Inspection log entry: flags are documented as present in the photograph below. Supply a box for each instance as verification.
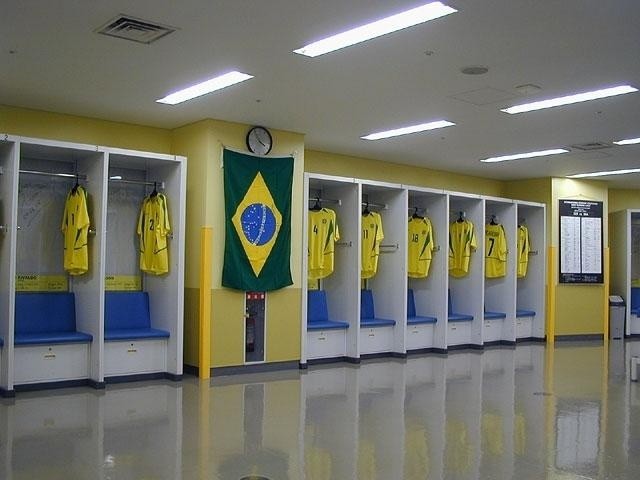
[221,146,294,292]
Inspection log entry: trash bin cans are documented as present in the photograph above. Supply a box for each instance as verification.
[609,295,625,340]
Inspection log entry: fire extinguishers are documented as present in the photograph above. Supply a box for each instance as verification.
[246,312,255,352]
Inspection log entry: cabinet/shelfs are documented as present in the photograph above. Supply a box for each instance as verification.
[609,209,640,337]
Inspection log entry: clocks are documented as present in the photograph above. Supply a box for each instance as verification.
[246,126,273,155]
